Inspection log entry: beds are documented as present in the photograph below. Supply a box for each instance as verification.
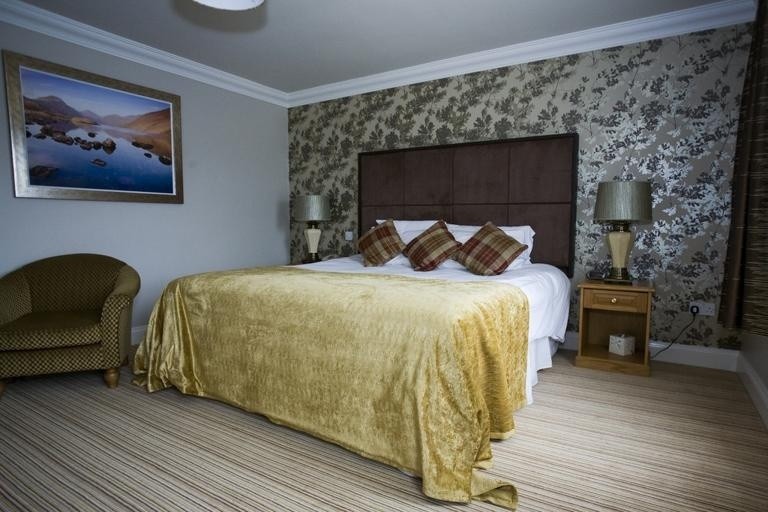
[135,131,579,508]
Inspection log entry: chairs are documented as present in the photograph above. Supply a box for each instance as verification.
[0,252,141,388]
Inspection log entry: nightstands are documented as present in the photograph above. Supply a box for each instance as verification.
[575,278,655,375]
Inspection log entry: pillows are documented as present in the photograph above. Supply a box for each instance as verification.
[357,218,405,268]
[451,221,527,277]
[375,219,446,268]
[437,223,536,272]
[403,219,461,271]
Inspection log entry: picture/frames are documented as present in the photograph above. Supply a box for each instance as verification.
[3,49,183,204]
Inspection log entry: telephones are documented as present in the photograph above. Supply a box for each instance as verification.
[322,254,338,261]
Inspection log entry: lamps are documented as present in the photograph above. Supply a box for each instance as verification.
[294,196,331,263]
[593,182,651,284]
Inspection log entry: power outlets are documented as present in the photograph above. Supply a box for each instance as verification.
[689,300,716,317]
